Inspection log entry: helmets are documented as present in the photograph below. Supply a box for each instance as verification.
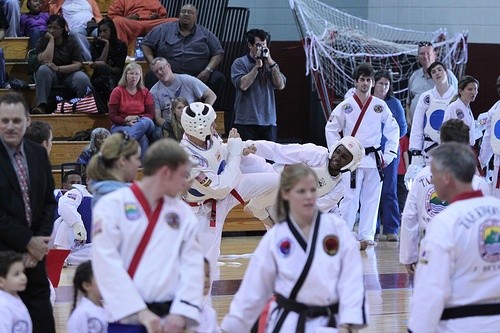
[181,101,217,141]
[329,136,365,172]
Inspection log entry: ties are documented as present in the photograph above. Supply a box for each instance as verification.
[15,152,32,229]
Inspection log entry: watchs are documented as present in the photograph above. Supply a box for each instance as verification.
[205,66,215,73]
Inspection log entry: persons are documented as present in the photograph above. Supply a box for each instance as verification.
[324,63,400,253]
[67,260,109,333]
[230,29,287,163]
[0,0,500,302]
[408,141,500,333]
[219,163,369,333]
[0,89,58,333]
[90,137,205,333]
[28,14,94,114]
[140,4,226,112]
[0,251,34,333]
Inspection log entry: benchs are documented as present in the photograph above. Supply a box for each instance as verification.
[0,0,266,233]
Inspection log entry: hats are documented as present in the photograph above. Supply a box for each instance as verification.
[438,34,445,39]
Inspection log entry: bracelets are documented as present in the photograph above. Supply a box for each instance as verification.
[269,62,276,69]
[56,66,60,73]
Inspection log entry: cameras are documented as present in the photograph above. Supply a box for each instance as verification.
[260,46,269,58]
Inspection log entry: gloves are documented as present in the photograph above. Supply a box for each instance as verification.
[411,155,425,174]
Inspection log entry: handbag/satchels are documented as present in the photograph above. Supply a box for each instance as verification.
[52,92,99,114]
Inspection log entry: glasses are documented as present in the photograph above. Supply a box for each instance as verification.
[126,72,142,76]
[118,130,130,142]
[419,42,432,47]
[153,64,168,75]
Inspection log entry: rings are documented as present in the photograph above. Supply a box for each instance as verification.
[30,261,34,266]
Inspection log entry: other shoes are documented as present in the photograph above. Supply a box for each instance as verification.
[387,233,398,241]
[31,104,47,114]
[374,234,381,241]
